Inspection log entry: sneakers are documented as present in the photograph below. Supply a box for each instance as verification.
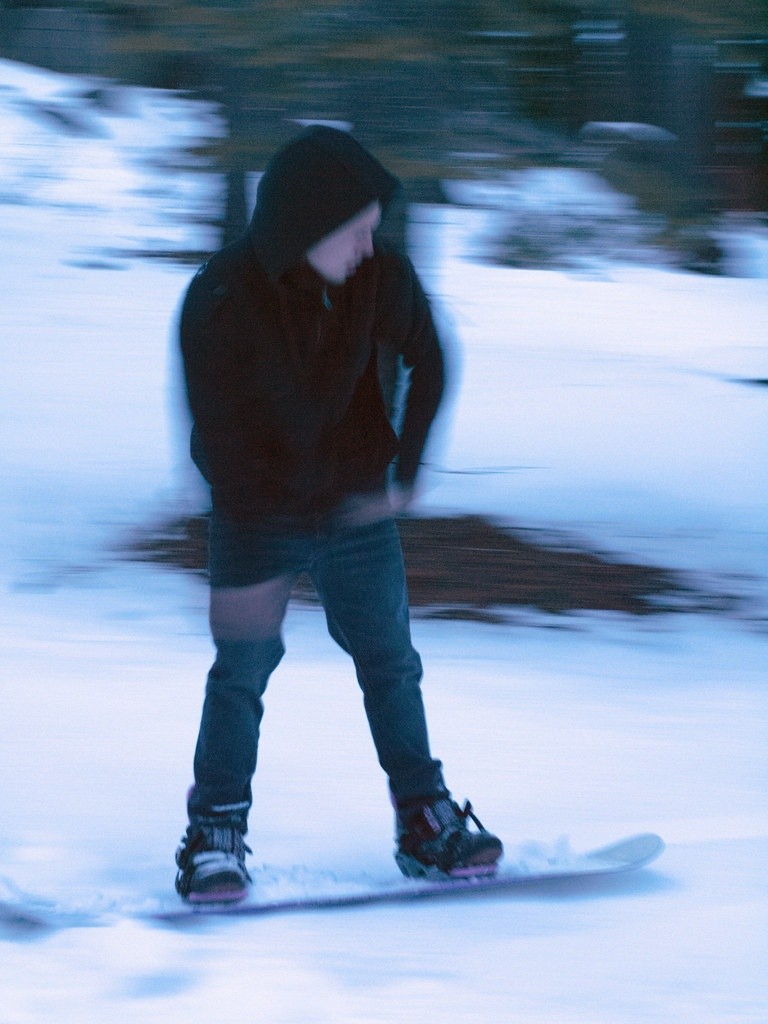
[394,798,503,882]
[176,809,254,906]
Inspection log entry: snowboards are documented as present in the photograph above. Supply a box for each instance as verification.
[0,833,666,927]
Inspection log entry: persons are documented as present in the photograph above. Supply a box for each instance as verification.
[175,122,503,905]
